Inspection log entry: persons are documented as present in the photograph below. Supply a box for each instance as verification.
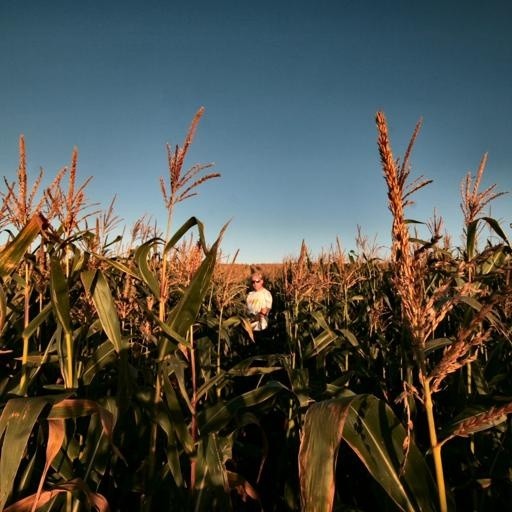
[246,272,274,359]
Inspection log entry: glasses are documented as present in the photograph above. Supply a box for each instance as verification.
[252,280,260,283]
